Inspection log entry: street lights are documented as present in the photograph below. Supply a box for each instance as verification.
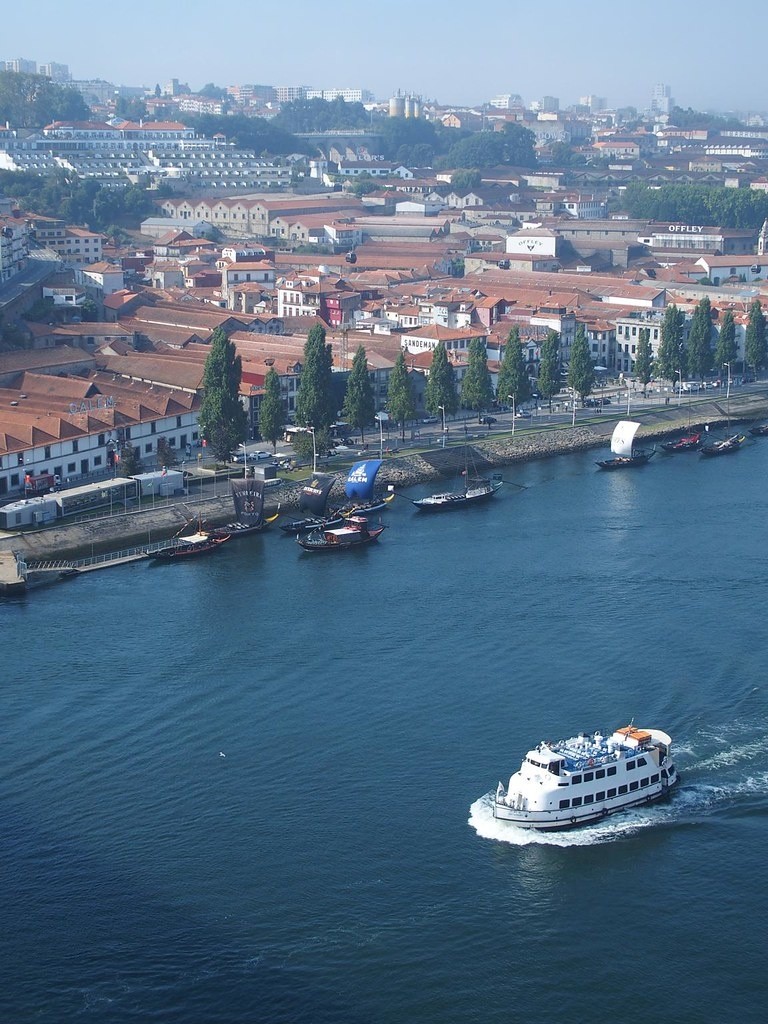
[674,369,682,407]
[374,416,382,458]
[182,461,189,502]
[568,386,576,426]
[307,430,316,473]
[507,393,515,436]
[162,465,169,507]
[438,405,445,431]
[238,442,247,482]
[198,424,206,453]
[109,439,120,479]
[724,362,731,399]
[19,458,30,500]
[623,377,630,416]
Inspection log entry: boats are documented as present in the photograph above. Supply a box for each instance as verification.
[700,432,746,456]
[748,418,768,437]
[491,717,679,831]
[661,434,705,452]
[295,512,386,552]
[146,509,233,561]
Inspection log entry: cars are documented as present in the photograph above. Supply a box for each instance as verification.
[421,415,438,424]
[481,417,497,424]
[517,410,532,418]
[598,397,611,405]
[685,374,753,391]
[584,400,601,407]
[229,451,251,462]
[250,450,272,459]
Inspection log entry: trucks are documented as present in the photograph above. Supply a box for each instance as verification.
[24,474,55,493]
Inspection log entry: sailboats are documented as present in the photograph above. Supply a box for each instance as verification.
[596,420,656,470]
[282,475,358,533]
[336,460,393,514]
[411,420,504,510]
[197,478,281,534]
